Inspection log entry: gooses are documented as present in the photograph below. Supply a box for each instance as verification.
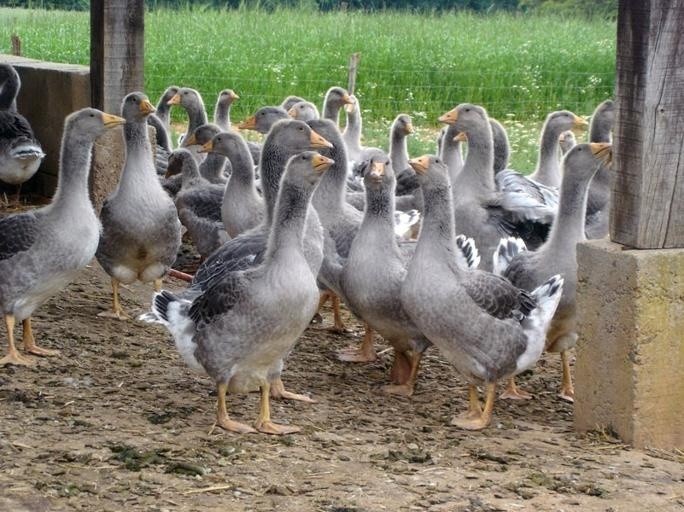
[99,87,616,436]
[0,106,127,367]
[0,62,47,212]
